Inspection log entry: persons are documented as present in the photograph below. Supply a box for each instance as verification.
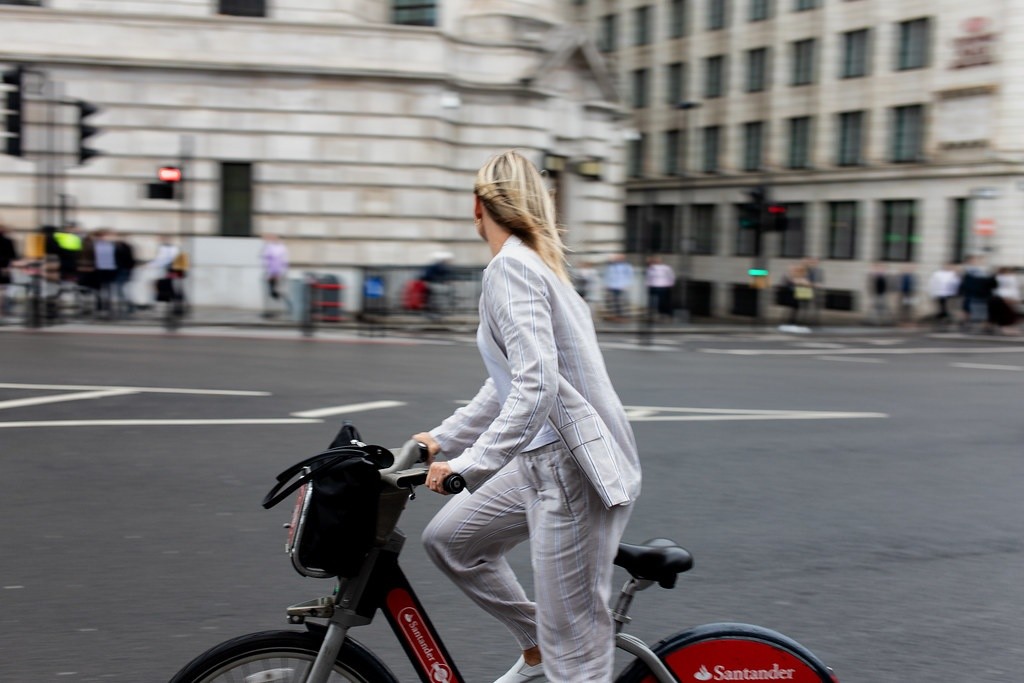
[255,226,288,325]
[0,217,135,326]
[602,253,633,317]
[419,249,455,315]
[647,254,676,323]
[414,152,644,683]
[864,251,1000,332]
[783,255,825,335]
[150,232,189,326]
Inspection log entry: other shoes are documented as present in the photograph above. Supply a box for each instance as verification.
[492,653,548,683]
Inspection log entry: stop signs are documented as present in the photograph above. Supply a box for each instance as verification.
[976,219,995,236]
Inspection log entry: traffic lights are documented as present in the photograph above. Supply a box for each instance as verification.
[0,70,24,156]
[76,101,101,167]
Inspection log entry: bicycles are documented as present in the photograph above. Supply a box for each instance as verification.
[167,425,841,683]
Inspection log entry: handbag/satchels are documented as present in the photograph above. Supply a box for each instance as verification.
[262,424,396,569]
